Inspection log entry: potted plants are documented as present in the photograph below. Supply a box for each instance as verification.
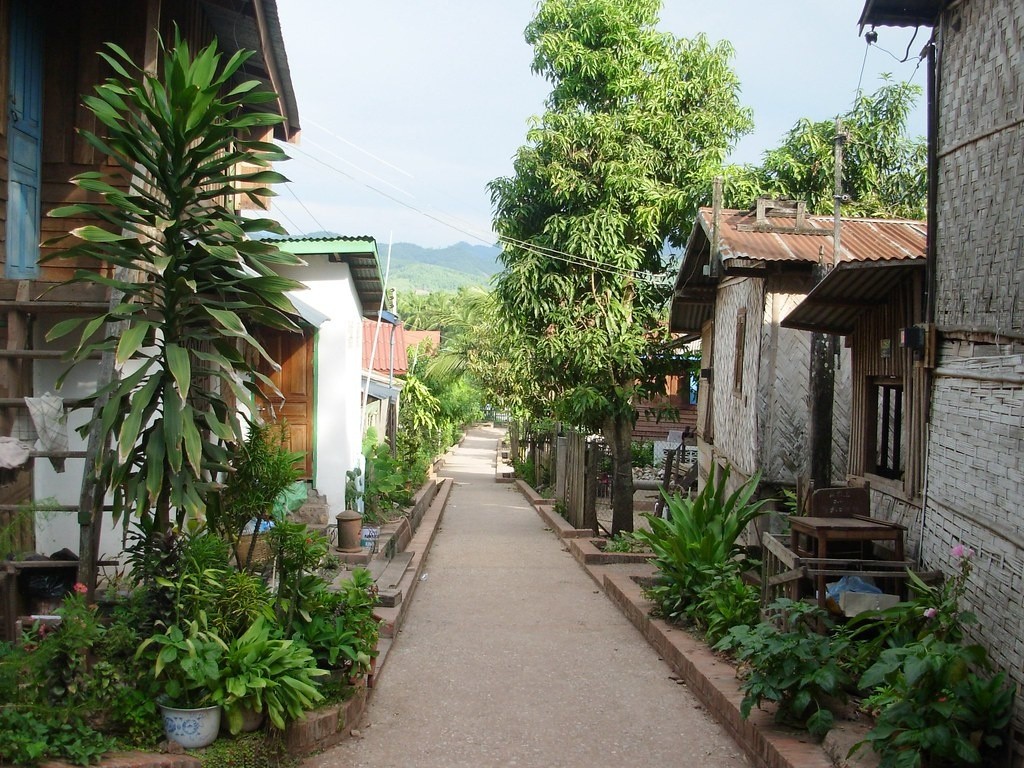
[222,610,331,732]
[305,623,361,691]
[135,553,231,749]
